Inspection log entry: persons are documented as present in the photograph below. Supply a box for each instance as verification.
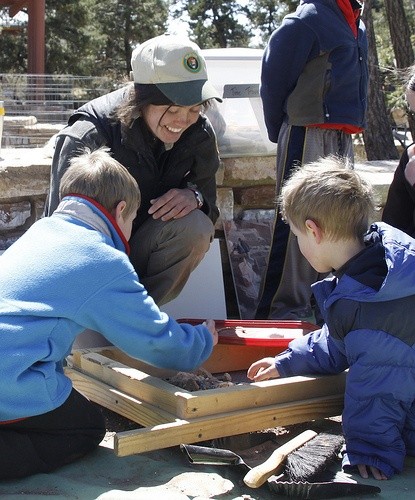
[247,154,415,480]
[41,32,224,351]
[0,145,218,483]
[253,0,369,320]
[381,65,415,240]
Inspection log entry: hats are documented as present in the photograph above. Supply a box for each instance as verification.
[130,33,226,106]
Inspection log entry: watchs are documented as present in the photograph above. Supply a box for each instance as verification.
[191,189,204,209]
